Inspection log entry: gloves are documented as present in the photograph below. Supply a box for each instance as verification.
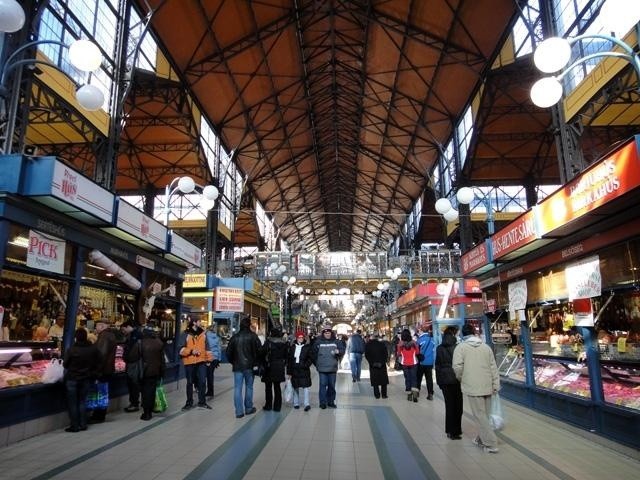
[212,359,221,368]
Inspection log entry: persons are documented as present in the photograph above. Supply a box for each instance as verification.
[226,317,437,418]
[30,312,165,433]
[436,323,502,453]
[177,315,221,410]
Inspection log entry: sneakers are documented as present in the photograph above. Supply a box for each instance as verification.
[236,414,244,418]
[484,446,499,453]
[304,405,310,411]
[472,437,484,447]
[427,393,433,400]
[382,395,388,398]
[376,395,380,399]
[295,406,299,409]
[125,406,139,412]
[182,404,192,411]
[141,412,153,420]
[198,402,212,410]
[246,407,256,414]
[206,393,214,396]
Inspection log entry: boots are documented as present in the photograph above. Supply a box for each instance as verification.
[406,391,412,401]
[411,387,419,401]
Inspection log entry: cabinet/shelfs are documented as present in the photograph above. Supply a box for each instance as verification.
[494,341,640,451]
[0,340,126,391]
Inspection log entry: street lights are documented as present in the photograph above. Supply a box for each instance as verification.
[270,262,304,296]
[529,0,640,109]
[0,0,104,111]
[372,267,402,300]
[163,175,219,227]
[434,185,495,237]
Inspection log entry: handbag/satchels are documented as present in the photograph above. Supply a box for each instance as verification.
[283,379,293,407]
[416,354,425,361]
[84,381,108,409]
[127,359,143,380]
[153,385,168,413]
[250,357,267,376]
[394,355,403,370]
[437,368,457,385]
[264,350,271,368]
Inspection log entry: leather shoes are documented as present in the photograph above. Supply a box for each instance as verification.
[320,404,326,409]
[263,404,272,410]
[274,406,281,412]
[447,432,452,438]
[80,424,87,430]
[328,402,336,407]
[452,435,462,440]
[65,426,80,432]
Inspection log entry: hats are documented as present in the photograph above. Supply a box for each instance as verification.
[143,324,153,333]
[323,325,331,333]
[94,318,108,323]
[271,329,285,337]
[295,331,305,337]
